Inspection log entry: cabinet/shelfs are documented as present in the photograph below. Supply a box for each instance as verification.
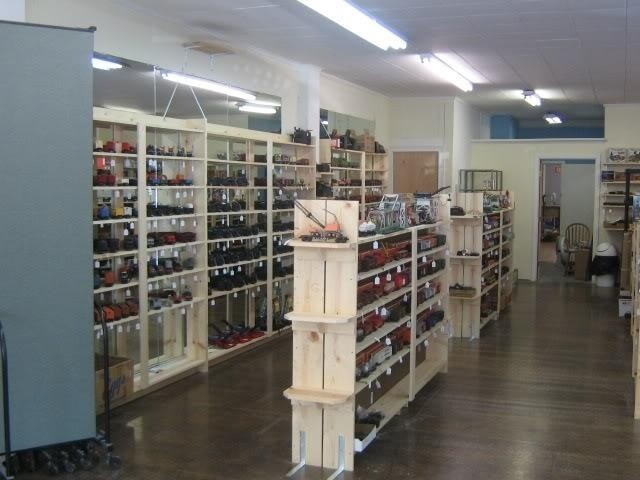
[602,160,640,231]
[94,107,517,480]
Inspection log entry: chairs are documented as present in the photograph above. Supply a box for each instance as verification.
[563,223,592,277]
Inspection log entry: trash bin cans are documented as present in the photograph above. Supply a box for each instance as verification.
[595,242,619,288]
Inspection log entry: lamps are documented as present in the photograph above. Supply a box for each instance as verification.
[421,52,474,94]
[159,70,257,102]
[521,90,563,124]
[92,56,122,71]
[299,0,409,53]
